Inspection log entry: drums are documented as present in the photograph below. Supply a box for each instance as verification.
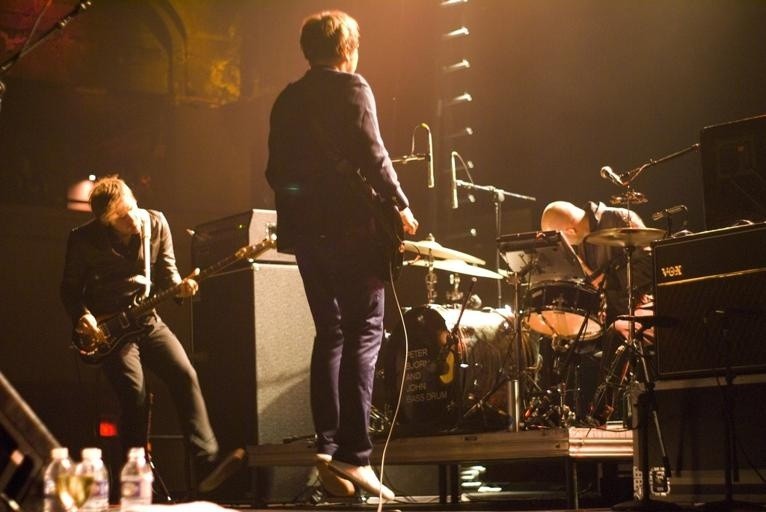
[520,279,609,339]
[384,305,539,432]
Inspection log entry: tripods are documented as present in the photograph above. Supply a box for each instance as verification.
[449,263,558,434]
[584,246,649,426]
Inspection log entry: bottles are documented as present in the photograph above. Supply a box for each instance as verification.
[78,449,110,510]
[118,448,153,510]
[45,445,73,511]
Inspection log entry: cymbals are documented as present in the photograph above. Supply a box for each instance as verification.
[585,229,667,245]
[402,241,486,265]
[403,259,502,280]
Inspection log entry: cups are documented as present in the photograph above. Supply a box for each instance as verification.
[59,475,92,512]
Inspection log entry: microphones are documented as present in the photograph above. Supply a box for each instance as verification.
[652,204,682,221]
[449,158,457,209]
[425,132,435,188]
[600,166,623,189]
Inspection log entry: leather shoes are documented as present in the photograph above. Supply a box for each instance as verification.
[198,448,246,493]
[311,453,395,500]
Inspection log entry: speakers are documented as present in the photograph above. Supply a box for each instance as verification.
[188,262,317,445]
[652,221,766,378]
[700,115,766,229]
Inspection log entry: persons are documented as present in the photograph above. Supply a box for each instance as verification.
[540,202,652,426]
[62,177,228,497]
[267,10,418,502]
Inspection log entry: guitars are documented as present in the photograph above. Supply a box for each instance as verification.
[71,236,277,364]
